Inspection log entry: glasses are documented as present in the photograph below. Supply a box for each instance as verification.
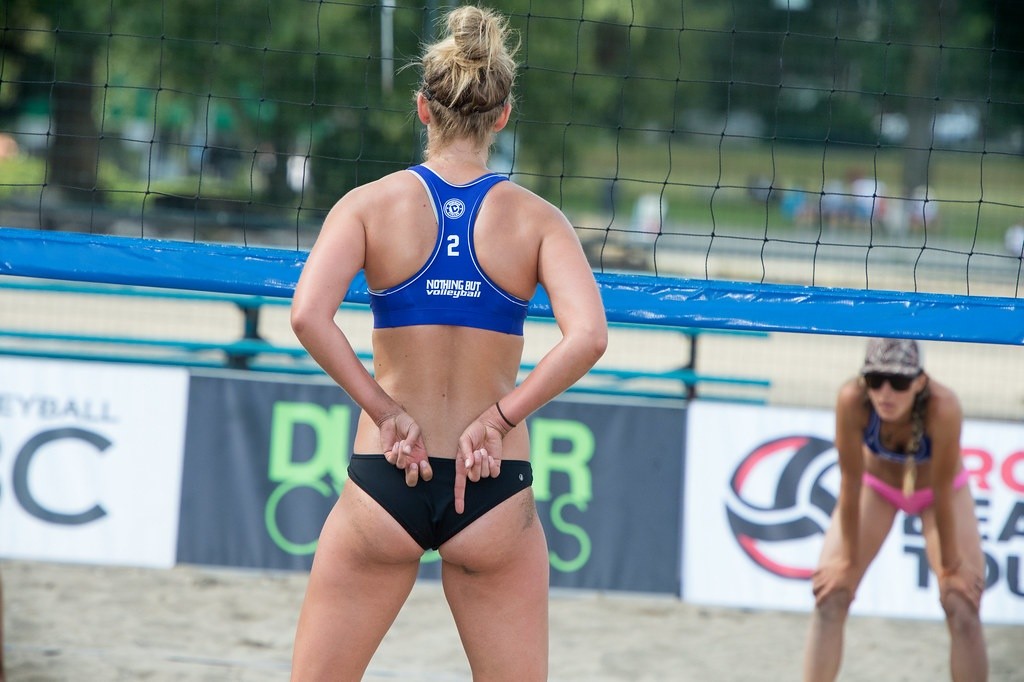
[864,367,923,392]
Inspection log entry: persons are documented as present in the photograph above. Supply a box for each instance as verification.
[634,188,669,248]
[749,168,942,238]
[802,337,989,682]
[288,0,609,680]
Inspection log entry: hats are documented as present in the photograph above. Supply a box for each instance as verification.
[861,337,921,376]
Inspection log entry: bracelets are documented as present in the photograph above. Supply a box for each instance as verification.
[496,401,520,430]
